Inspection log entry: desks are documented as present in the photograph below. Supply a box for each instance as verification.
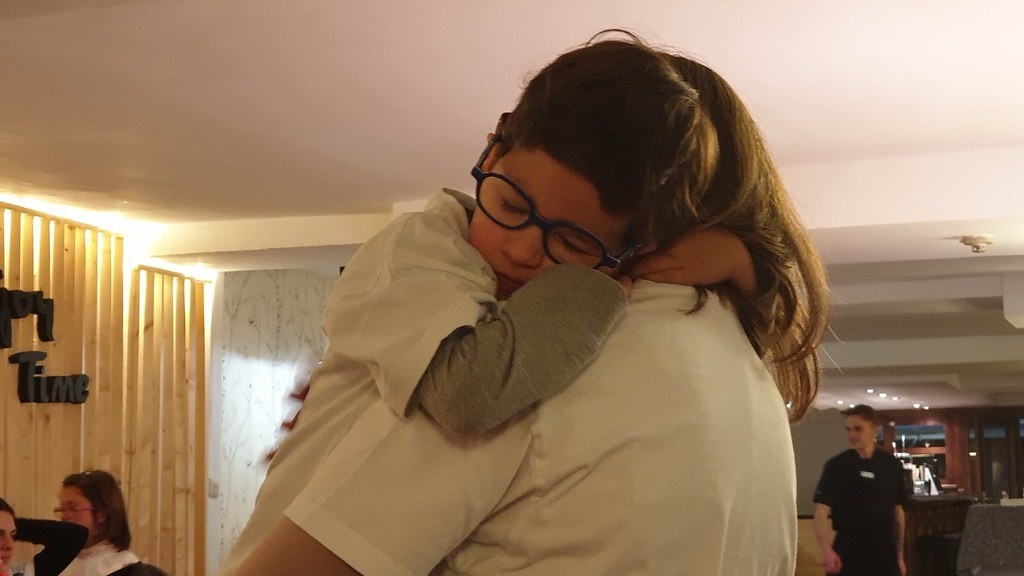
[916,531,963,576]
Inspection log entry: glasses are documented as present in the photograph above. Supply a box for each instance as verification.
[469,130,643,271]
[52,505,95,518]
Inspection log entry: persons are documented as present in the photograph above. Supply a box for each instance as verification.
[224,41,830,574]
[813,404,907,576]
[1,468,168,576]
